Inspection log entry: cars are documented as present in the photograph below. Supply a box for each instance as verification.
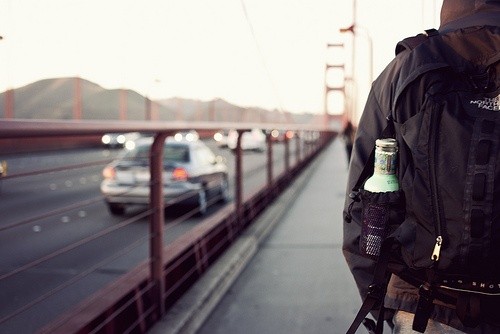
[100,134,229,218]
[214,129,321,149]
[101,131,140,148]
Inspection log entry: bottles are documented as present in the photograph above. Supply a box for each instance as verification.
[359,138,401,260]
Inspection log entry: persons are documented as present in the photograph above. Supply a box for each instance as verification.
[342,0,500,334]
[342,122,357,160]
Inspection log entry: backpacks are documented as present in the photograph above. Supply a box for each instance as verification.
[393,31,500,316]
